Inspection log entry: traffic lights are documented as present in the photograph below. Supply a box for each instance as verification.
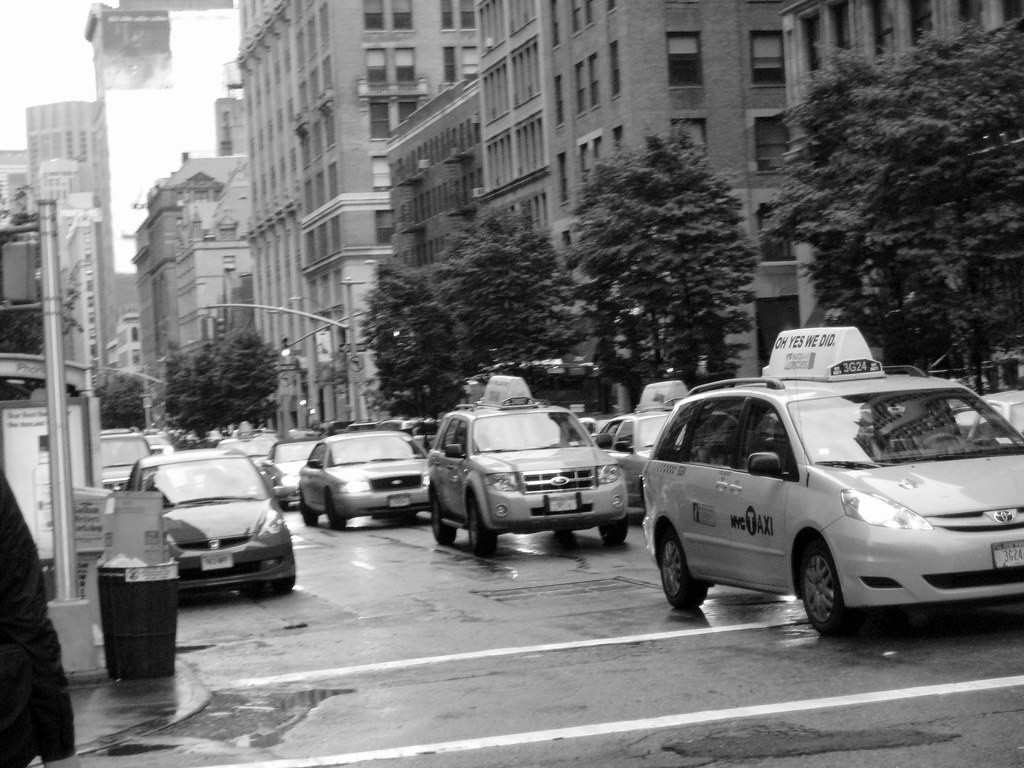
[213,316,227,342]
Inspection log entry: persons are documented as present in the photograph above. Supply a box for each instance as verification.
[0,472,78,768]
[698,416,738,464]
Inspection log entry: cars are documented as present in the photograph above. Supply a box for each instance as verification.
[127,446,296,602]
[146,380,1024,510]
[426,376,629,558]
[300,430,429,530]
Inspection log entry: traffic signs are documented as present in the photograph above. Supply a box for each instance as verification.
[338,343,367,352]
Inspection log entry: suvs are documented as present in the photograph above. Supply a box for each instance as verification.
[101,426,162,494]
[644,327,1024,638]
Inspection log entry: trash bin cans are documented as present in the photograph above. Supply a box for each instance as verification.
[96,561,180,683]
